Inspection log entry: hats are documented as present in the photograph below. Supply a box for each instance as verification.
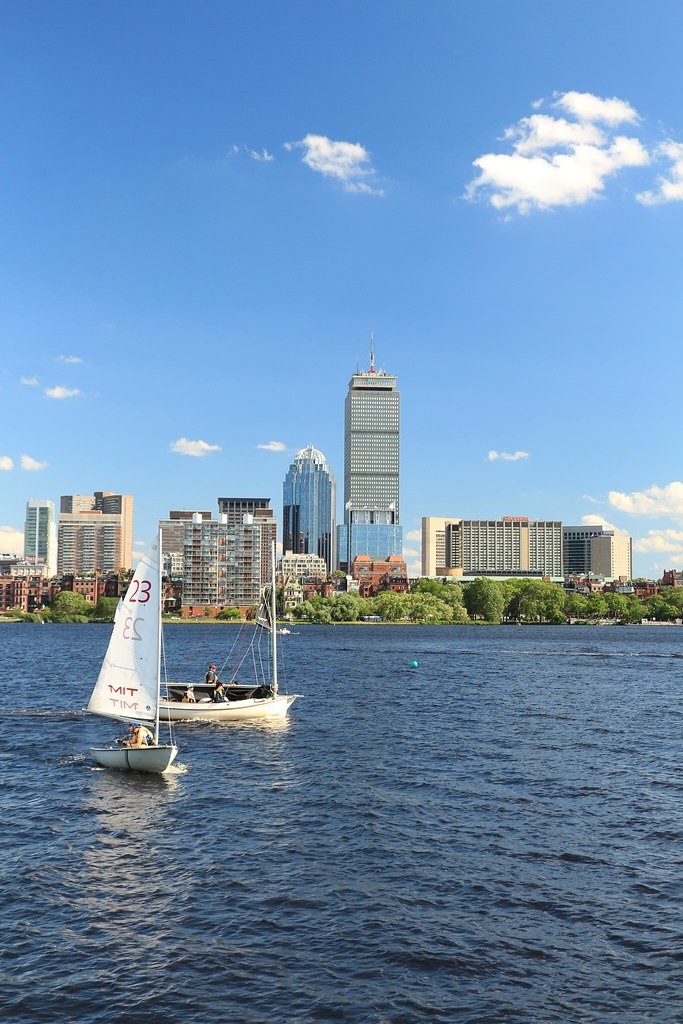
[133,723,138,727]
[187,684,194,688]
[210,665,216,669]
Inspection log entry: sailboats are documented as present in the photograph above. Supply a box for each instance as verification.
[158,540,305,721]
[80,531,178,773]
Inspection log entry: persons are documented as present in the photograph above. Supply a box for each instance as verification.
[204,665,220,683]
[181,683,196,703]
[121,721,155,747]
[212,682,229,703]
